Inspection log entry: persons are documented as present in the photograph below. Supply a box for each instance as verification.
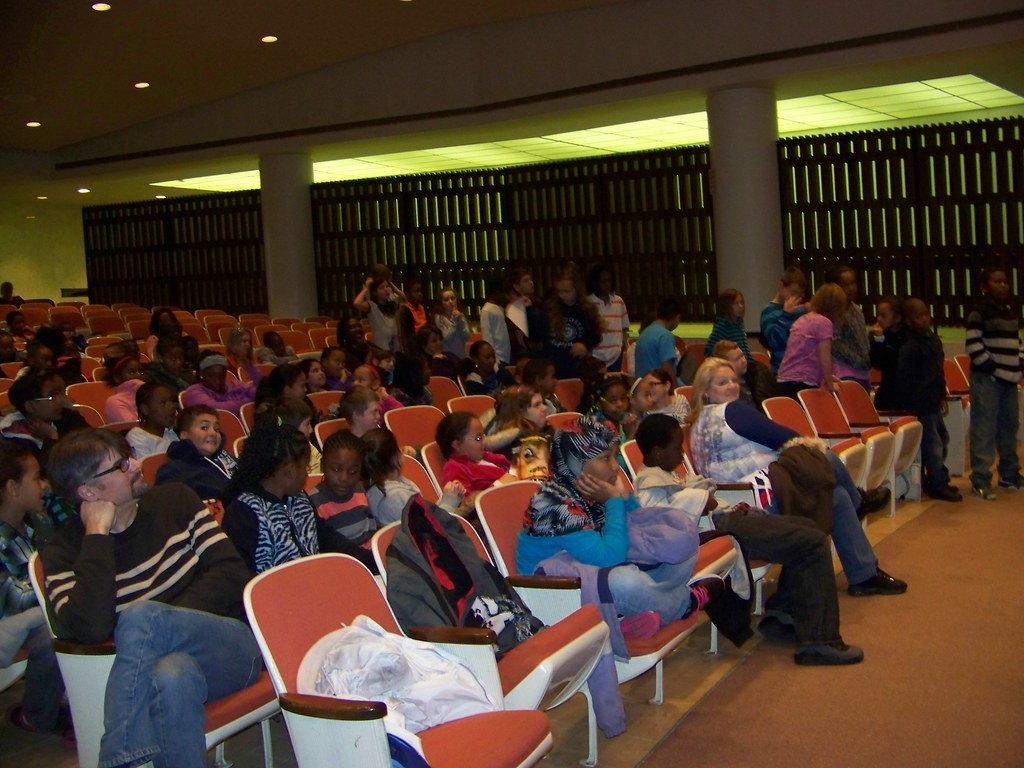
[0,263,945,768]
[965,262,1024,501]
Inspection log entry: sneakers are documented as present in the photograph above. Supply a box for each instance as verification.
[619,608,660,641]
[682,573,725,620]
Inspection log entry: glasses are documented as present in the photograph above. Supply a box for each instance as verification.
[34,386,68,400]
[649,380,663,387]
[466,432,487,442]
[92,446,137,479]
[232,327,244,335]
[297,462,313,474]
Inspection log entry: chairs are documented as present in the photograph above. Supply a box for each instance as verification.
[0,290,922,768]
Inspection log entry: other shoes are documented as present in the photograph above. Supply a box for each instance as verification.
[757,615,802,642]
[998,473,1024,492]
[927,480,962,502]
[794,640,864,665]
[923,479,960,494]
[848,566,907,595]
[856,485,891,521]
[971,485,996,499]
